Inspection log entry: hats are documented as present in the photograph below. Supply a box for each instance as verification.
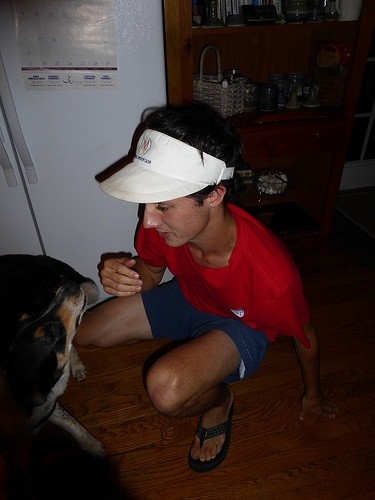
[98,128,234,203]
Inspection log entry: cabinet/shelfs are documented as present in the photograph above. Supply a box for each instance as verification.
[163,0,375,251]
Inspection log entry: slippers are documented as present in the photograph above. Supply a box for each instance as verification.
[186,382,233,474]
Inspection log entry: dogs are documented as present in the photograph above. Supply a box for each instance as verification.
[0,254,106,461]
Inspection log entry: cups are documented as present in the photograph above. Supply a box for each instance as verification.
[335,0,363,21]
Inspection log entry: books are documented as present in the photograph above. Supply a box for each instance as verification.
[205,0,282,23]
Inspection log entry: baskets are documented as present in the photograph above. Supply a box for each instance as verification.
[191,45,244,120]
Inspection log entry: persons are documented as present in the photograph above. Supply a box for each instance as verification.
[74,102,338,471]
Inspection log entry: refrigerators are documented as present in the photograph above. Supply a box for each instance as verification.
[0,0,175,310]
